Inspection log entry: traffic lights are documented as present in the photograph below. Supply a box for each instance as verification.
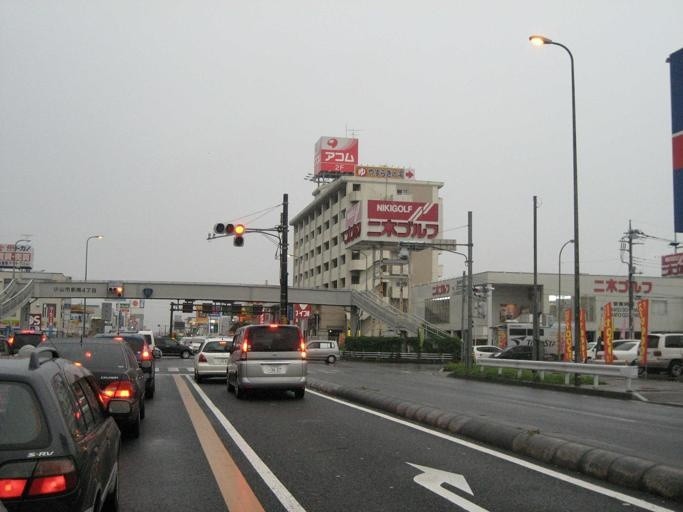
[211,222,247,248]
[107,287,122,297]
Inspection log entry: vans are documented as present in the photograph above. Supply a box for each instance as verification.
[222,321,310,402]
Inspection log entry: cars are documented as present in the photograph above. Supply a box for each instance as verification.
[0,320,238,400]
[464,334,643,378]
[302,339,341,364]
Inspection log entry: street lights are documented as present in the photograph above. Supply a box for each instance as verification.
[557,239,574,361]
[12,238,33,280]
[392,240,472,369]
[81,234,102,338]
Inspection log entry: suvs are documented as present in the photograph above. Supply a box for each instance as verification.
[635,328,682,379]
[36,331,148,442]
[0,345,123,512]
